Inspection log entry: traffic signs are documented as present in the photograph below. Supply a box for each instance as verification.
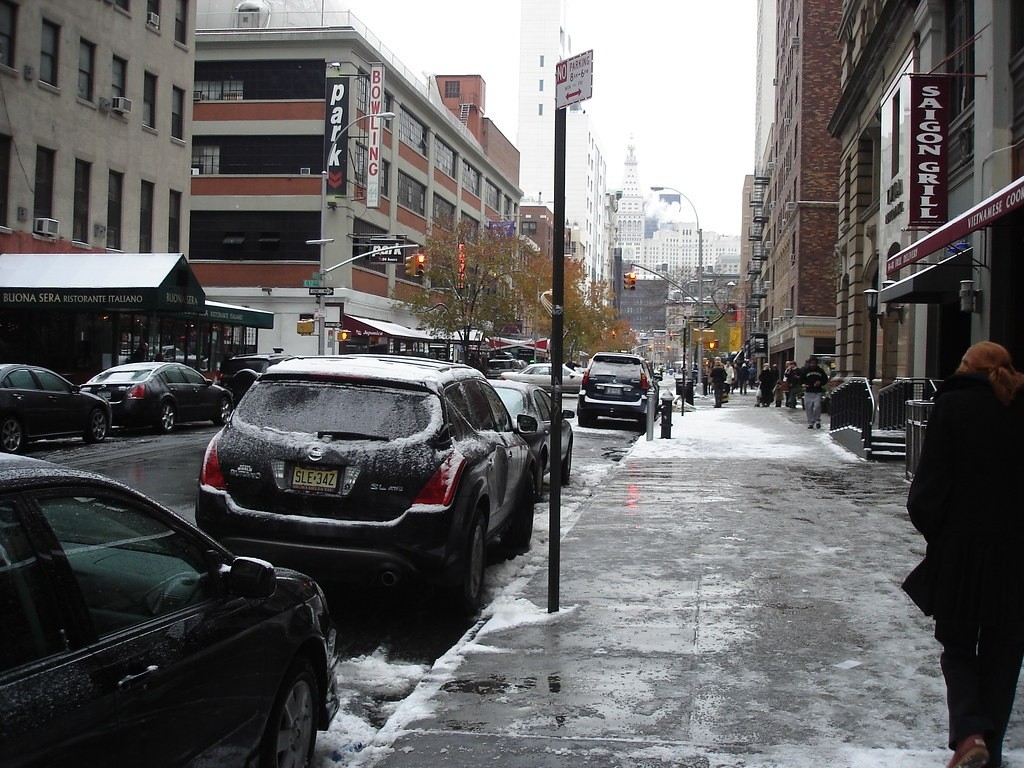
[689,316,710,323]
[309,288,342,328]
[556,49,593,109]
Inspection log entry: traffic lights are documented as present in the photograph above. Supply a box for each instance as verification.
[629,271,635,290]
[414,254,426,277]
[624,273,629,289]
[341,331,350,340]
[405,256,414,276]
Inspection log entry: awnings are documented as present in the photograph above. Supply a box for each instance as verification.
[886,174,1024,277]
[343,313,436,341]
[877,247,973,304]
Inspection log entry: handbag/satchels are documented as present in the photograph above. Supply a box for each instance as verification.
[755,389,762,399]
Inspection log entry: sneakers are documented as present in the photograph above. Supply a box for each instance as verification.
[945,735,990,768]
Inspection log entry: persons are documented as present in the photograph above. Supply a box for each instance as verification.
[705,356,809,407]
[658,361,699,387]
[902,340,1024,768]
[133,342,148,362]
[799,356,828,429]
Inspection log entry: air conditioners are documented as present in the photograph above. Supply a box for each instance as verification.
[112,96,133,114]
[300,167,311,176]
[789,37,799,49]
[783,118,791,127]
[784,76,790,84]
[768,203,773,210]
[148,11,160,26]
[782,309,794,318]
[784,202,795,213]
[789,254,795,262]
[772,78,778,86]
[762,321,770,330]
[772,200,777,208]
[193,91,203,101]
[765,241,772,249]
[34,217,60,236]
[764,280,771,289]
[766,162,774,170]
[772,318,779,326]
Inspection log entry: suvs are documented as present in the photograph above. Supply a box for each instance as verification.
[218,354,293,409]
[195,354,539,618]
[576,351,662,429]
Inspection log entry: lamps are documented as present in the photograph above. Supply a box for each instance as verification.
[959,280,982,314]
[327,201,338,212]
[882,275,903,325]
[863,288,884,328]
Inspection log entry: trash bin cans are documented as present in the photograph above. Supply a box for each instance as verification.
[676,378,694,405]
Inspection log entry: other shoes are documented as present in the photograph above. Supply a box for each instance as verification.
[816,424,821,428]
[808,425,813,429]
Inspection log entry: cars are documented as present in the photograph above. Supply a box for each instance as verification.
[78,361,234,434]
[0,449,339,768]
[0,364,113,453]
[486,377,573,491]
[652,369,662,380]
[502,363,583,394]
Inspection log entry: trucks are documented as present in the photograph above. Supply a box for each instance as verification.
[486,353,527,378]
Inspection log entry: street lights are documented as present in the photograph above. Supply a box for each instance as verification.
[651,187,703,383]
[318,113,395,354]
